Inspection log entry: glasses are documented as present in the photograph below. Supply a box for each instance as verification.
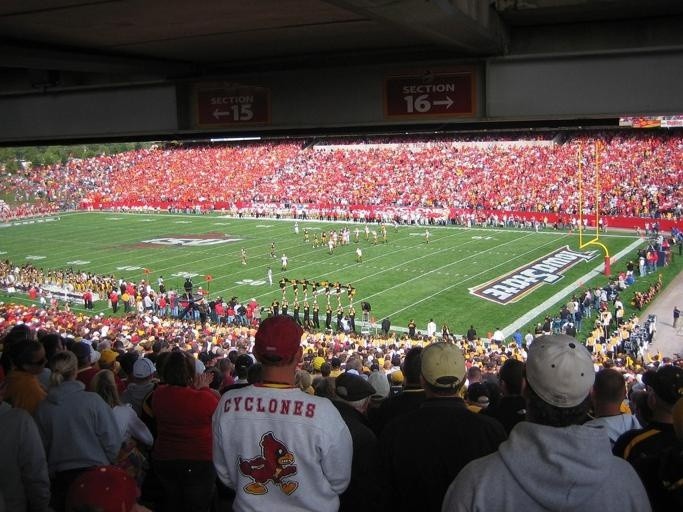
[29,360,47,366]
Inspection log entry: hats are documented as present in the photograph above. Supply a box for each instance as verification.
[254,316,304,365]
[100,349,119,364]
[313,357,325,370]
[526,334,595,407]
[69,467,142,512]
[133,358,156,379]
[390,371,404,382]
[463,382,490,402]
[336,374,376,401]
[422,342,467,388]
[366,369,390,401]
[642,365,683,403]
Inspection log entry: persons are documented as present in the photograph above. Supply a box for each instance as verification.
[237,227,433,268]
[624,232,682,259]
[0,134,683,230]
[3,259,682,512]
